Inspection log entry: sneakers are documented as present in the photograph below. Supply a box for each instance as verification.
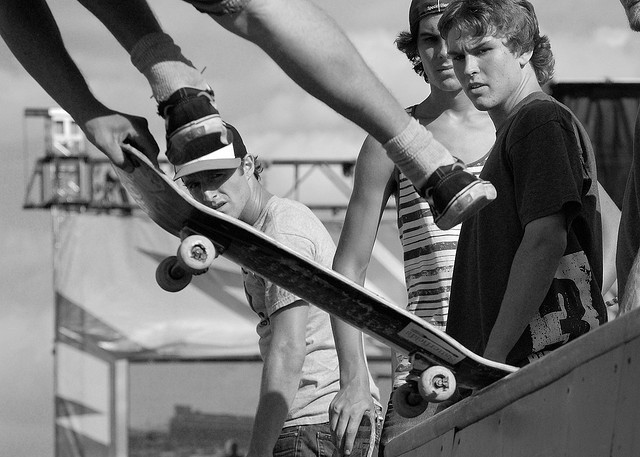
[414,157,497,230]
[155,87,229,165]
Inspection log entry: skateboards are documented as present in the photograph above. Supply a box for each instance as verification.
[107,143,522,420]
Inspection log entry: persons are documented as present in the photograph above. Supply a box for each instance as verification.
[328,0,623,456]
[616,0,640,318]
[434,0,609,418]
[0,0,497,231]
[172,119,383,457]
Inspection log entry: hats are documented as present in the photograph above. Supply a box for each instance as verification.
[173,124,248,181]
[409,0,453,28]
